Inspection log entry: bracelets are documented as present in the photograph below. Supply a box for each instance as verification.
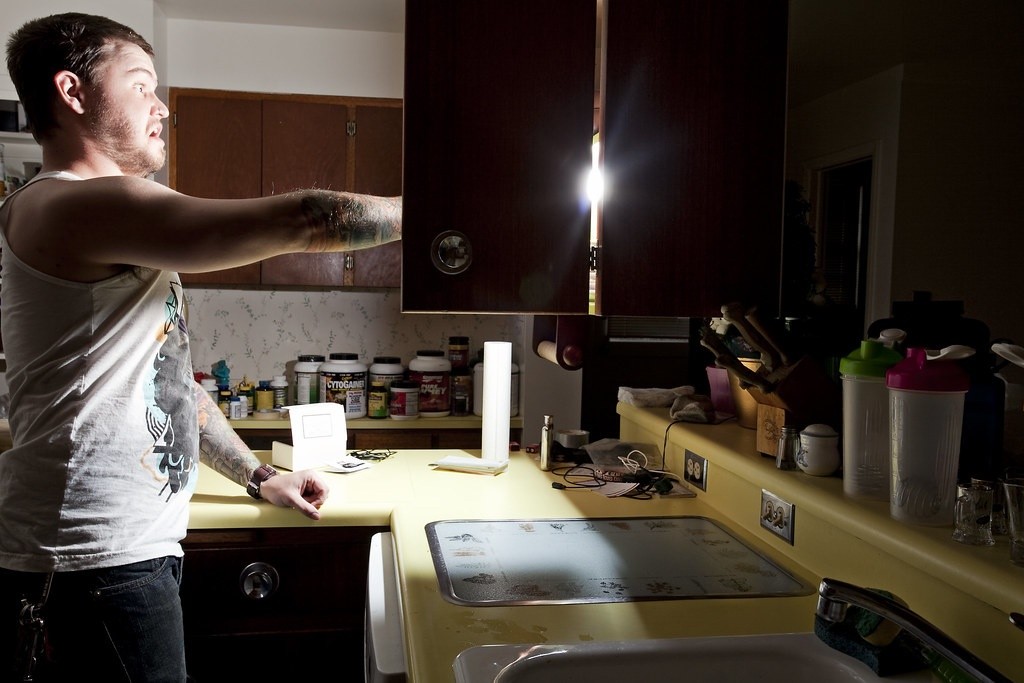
[246,463,280,500]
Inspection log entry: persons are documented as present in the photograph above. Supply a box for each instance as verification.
[0,12,402,683]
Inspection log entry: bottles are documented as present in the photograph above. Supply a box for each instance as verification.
[541,414,554,471]
[885,347,969,529]
[838,339,903,504]
[777,425,802,473]
[201,336,520,420]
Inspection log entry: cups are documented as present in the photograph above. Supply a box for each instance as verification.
[1003,478,1024,568]
[952,484,995,546]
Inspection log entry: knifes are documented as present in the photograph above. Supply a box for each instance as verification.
[697,300,804,391]
